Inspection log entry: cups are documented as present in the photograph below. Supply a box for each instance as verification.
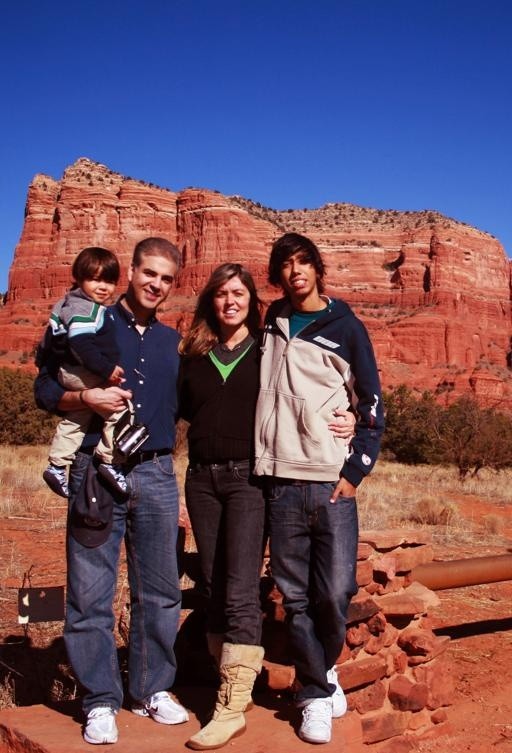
[112,422,151,463]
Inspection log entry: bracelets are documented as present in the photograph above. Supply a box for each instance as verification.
[79,389,85,404]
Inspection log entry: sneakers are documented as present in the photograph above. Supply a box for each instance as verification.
[298,695,335,745]
[82,704,121,746]
[131,688,191,725]
[328,663,351,721]
[41,466,72,500]
[96,459,130,494]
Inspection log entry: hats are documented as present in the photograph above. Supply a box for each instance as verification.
[68,461,115,549]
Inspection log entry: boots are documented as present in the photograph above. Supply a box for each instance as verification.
[184,631,267,750]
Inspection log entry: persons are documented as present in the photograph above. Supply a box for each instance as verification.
[41,245,131,500]
[246,231,385,748]
[31,236,191,748]
[173,261,361,752]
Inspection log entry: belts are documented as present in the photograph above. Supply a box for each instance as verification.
[78,442,174,465]
